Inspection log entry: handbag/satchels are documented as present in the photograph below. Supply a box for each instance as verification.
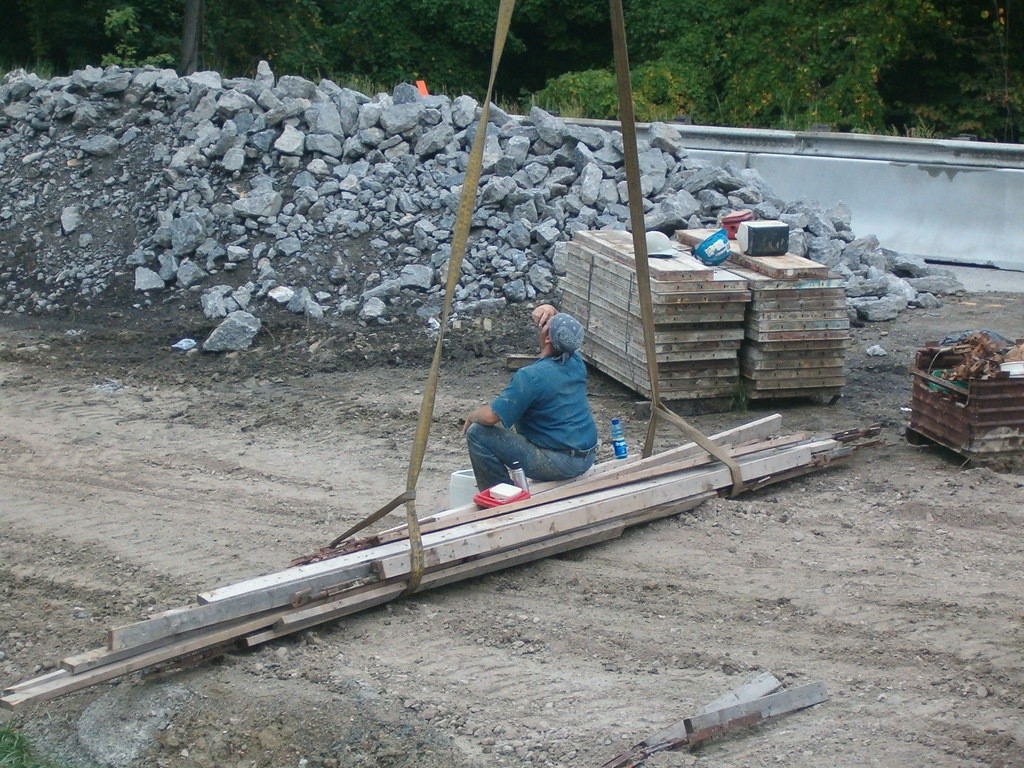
[723,210,752,239]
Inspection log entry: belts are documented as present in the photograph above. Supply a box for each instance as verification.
[554,449,598,457]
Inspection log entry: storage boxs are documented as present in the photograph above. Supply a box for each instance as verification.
[909,338,1024,459]
[474,486,531,508]
[722,210,753,238]
[490,482,523,500]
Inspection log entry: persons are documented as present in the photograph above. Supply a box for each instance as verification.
[460,303,598,495]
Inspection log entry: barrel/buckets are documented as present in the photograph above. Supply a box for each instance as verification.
[928,347,969,394]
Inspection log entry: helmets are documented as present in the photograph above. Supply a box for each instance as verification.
[691,228,731,266]
[645,231,679,257]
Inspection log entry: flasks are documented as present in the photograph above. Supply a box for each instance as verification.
[512,461,529,494]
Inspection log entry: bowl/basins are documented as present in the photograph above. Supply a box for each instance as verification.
[1000,361,1024,378]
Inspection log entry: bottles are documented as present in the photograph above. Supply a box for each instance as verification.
[611,419,627,458]
